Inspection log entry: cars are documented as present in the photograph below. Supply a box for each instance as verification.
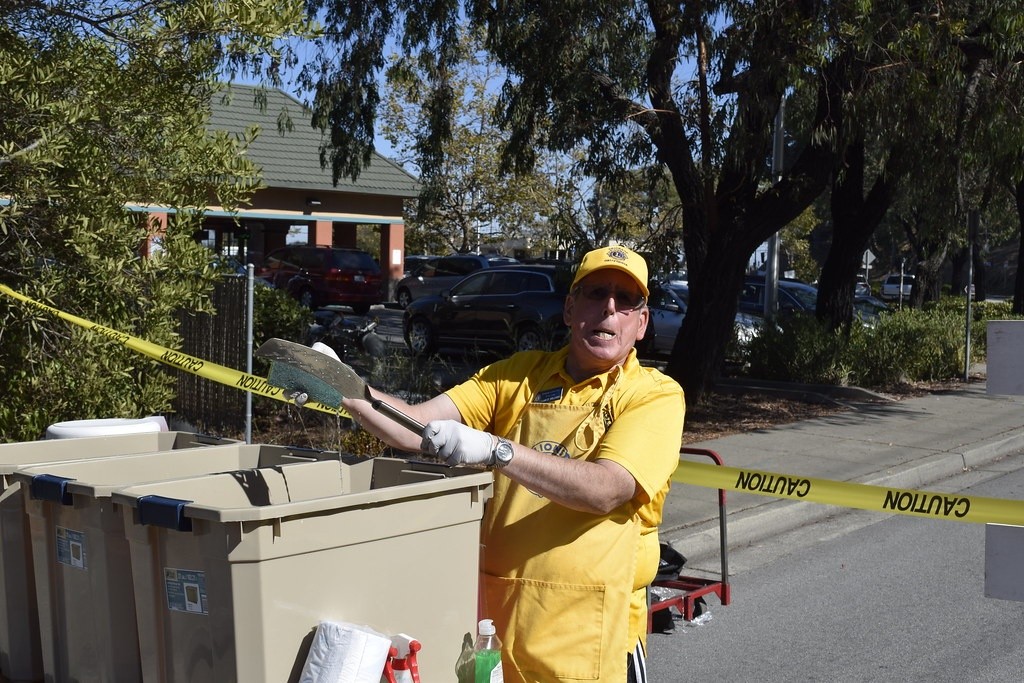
[638,279,783,361]
[199,250,301,323]
[624,252,920,332]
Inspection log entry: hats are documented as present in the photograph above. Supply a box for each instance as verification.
[569,246,650,305]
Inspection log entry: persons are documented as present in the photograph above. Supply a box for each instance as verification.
[282,245,685,682]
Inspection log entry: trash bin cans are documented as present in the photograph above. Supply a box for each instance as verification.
[13,443,371,681]
[0,429,248,683]
[112,450,495,683]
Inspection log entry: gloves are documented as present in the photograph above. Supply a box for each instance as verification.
[282,342,342,407]
[420,419,496,467]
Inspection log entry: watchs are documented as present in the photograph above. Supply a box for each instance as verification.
[488,436,514,468]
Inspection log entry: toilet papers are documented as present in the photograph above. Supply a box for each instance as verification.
[293,619,392,683]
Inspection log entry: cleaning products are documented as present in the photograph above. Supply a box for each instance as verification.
[468,618,508,683]
[384,631,424,683]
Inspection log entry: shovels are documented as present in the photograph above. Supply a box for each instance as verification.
[256,337,427,437]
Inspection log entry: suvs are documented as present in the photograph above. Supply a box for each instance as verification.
[268,242,384,315]
[389,261,582,363]
[395,252,573,310]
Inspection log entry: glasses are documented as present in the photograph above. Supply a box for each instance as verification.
[571,285,647,311]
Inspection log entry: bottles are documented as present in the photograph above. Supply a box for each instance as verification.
[389,633,422,683]
[473,619,504,682]
[383,646,398,683]
